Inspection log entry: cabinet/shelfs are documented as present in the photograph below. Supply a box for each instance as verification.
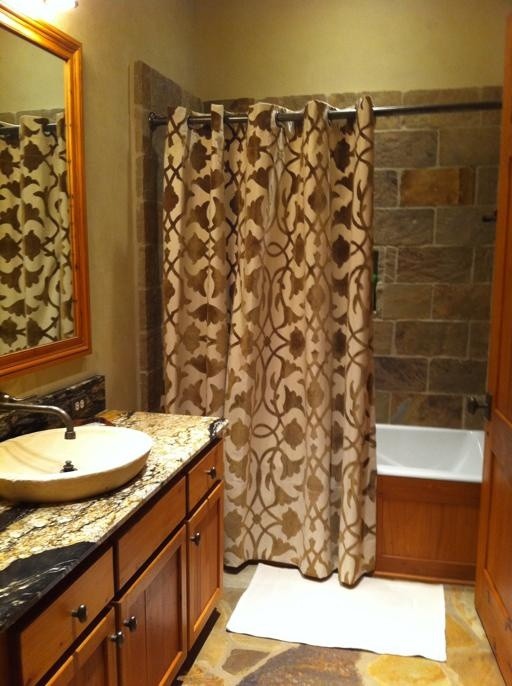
[186,432,231,662]
[2,475,190,685]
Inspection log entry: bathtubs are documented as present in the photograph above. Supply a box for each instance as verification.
[366,422,486,482]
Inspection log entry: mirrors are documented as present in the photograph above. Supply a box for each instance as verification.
[0,1,96,384]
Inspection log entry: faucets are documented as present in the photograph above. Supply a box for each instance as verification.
[0,393,77,440]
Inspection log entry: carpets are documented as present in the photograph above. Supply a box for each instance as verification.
[224,561,448,663]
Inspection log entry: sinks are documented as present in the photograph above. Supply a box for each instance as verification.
[0,425,155,504]
